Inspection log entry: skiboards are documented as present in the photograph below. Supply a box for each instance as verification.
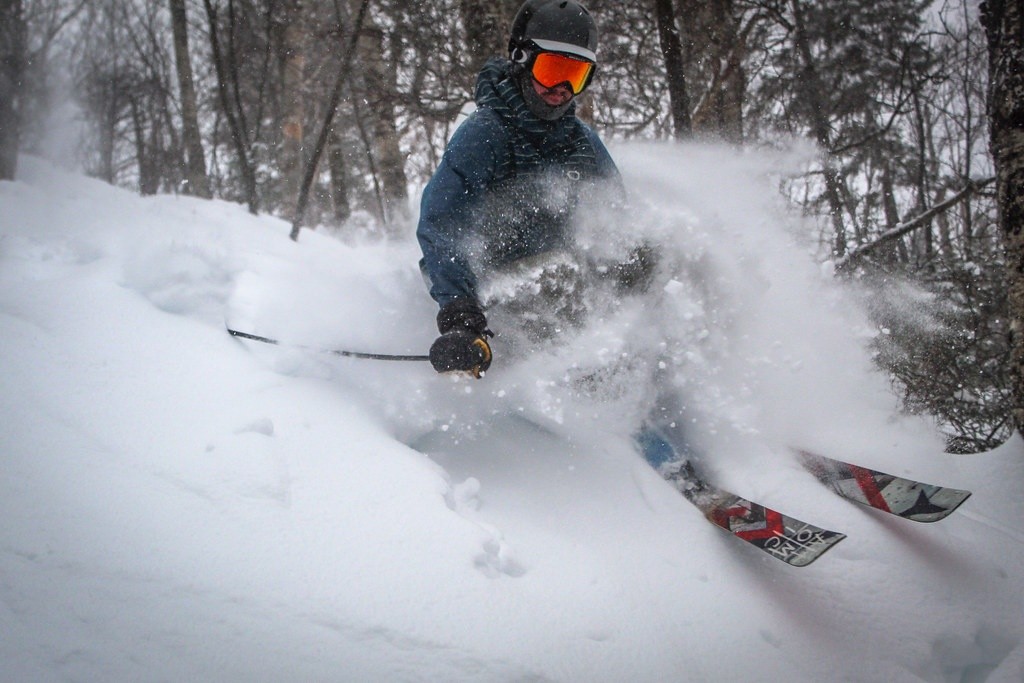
[686,442,974,567]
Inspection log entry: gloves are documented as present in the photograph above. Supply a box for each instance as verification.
[430,298,494,379]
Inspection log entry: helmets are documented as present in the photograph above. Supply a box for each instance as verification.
[507,0,598,63]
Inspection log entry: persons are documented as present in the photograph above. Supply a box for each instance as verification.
[417,1,662,373]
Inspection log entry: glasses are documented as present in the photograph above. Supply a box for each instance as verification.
[529,49,594,96]
